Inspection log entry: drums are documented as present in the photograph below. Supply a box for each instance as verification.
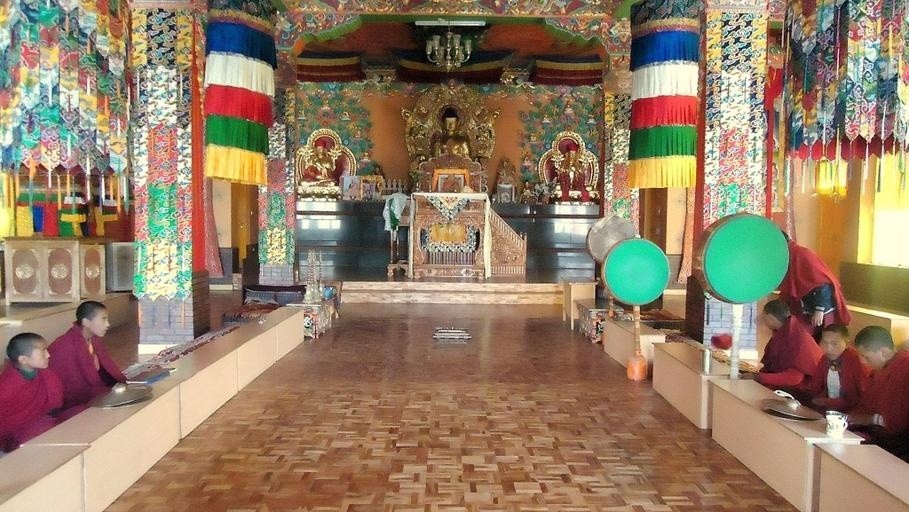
[601,238,670,306]
[689,211,790,305]
[586,214,636,265]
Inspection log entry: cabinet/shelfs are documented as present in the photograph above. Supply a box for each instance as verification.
[602,318,664,367]
[138,342,238,438]
[259,305,303,359]
[708,379,865,510]
[216,323,274,387]
[1,444,81,510]
[22,382,180,512]
[651,341,740,429]
[811,444,909,511]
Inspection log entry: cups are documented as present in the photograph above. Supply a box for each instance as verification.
[824,411,849,438]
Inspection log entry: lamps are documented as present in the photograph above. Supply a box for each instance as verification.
[426,19,473,72]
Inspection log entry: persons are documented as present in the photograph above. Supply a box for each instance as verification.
[741,230,909,450]
[429,108,472,163]
[559,143,589,201]
[0,301,147,452]
[298,139,335,186]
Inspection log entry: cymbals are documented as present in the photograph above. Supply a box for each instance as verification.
[88,381,153,407]
[760,398,823,419]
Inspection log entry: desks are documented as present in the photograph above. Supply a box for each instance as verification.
[561,275,623,344]
[286,279,342,339]
